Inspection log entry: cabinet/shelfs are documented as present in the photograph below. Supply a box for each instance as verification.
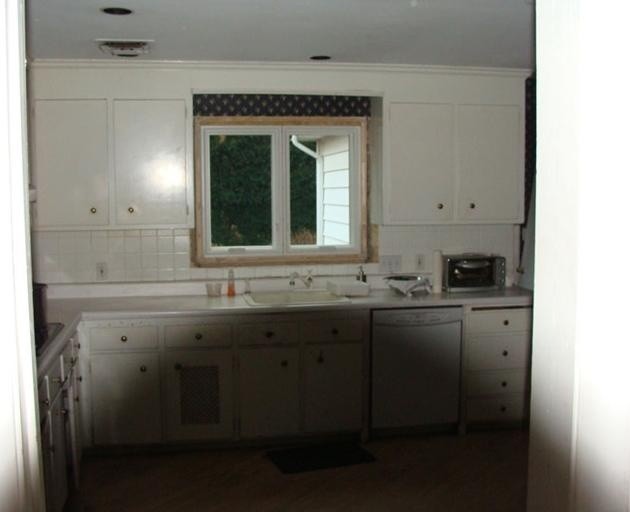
[34,98,186,224]
[371,103,521,219]
[239,318,364,437]
[467,306,530,421]
[39,332,86,512]
[90,324,235,447]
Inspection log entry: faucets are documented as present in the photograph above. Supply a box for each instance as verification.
[289,271,312,289]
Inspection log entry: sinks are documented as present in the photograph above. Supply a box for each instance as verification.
[242,290,352,306]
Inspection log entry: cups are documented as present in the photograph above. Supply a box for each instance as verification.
[206,282,222,297]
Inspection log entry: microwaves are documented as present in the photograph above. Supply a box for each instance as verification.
[443,254,506,294]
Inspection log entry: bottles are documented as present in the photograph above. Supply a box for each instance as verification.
[227,268,235,298]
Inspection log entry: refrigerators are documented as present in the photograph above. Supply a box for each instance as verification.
[371,307,462,426]
